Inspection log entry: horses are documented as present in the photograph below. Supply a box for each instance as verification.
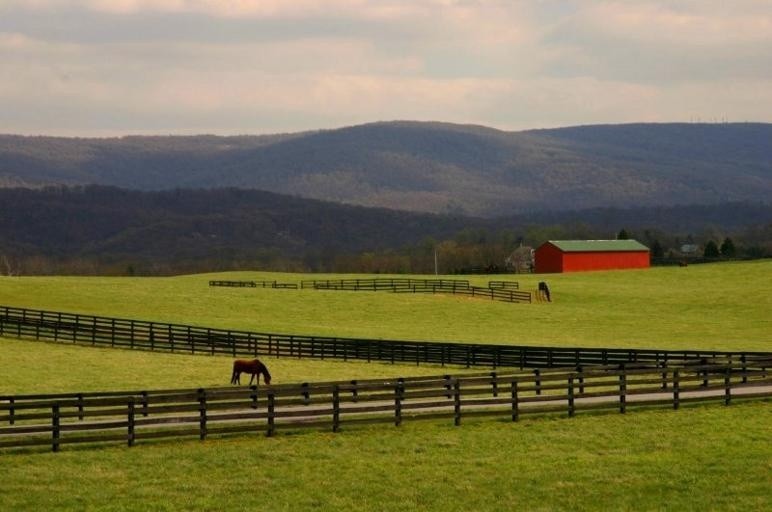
[538,281,552,303]
[231,358,272,386]
